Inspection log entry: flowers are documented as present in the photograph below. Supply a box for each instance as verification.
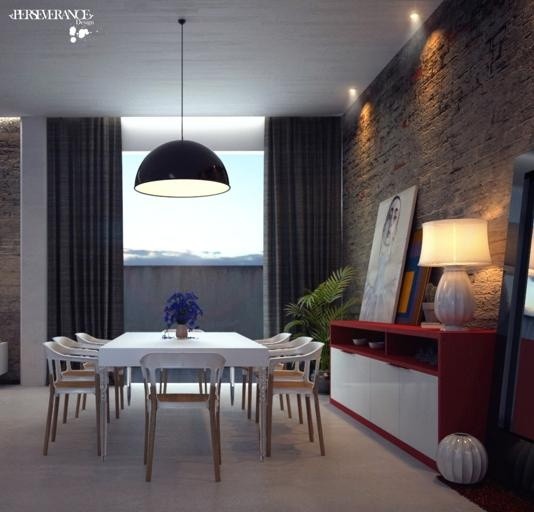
[160,290,204,330]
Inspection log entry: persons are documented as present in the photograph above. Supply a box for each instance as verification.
[358,194,403,322]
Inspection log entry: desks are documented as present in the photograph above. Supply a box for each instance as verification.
[97,331,268,460]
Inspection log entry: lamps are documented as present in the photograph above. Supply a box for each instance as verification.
[135,16,230,198]
[418,217,491,324]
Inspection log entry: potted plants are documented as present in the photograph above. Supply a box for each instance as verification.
[280,266,359,395]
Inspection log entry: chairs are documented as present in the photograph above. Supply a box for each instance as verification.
[242,335,326,458]
[162,329,206,394]
[141,353,226,484]
[41,333,127,456]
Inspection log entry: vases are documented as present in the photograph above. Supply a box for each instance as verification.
[174,324,189,338]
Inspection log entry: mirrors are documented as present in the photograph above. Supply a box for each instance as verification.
[490,151,534,443]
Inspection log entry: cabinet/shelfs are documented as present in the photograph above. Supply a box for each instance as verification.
[328,319,497,473]
